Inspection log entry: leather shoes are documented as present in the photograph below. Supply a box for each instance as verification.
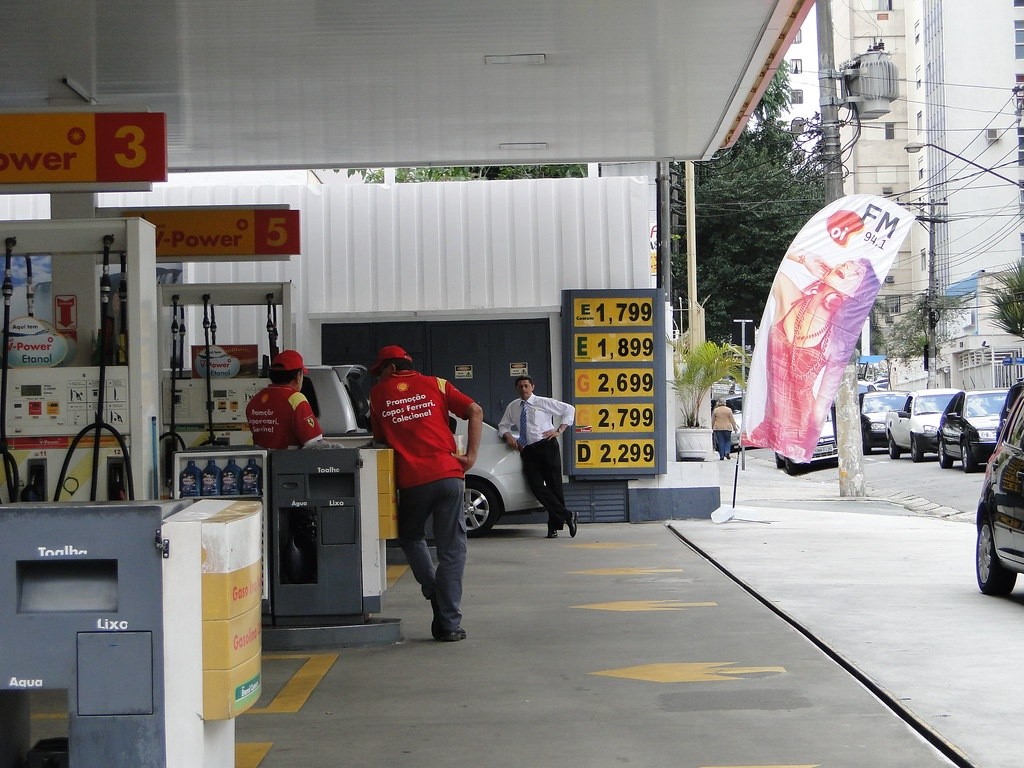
[431,605,468,642]
[546,528,558,538]
[566,510,577,538]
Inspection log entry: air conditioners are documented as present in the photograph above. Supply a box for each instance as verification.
[883,187,893,194]
[986,129,998,140]
[886,317,894,322]
[886,276,894,283]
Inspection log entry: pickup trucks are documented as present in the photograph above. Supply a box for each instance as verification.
[885,389,973,462]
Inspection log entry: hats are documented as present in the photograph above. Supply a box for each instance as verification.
[270,349,310,377]
[367,345,413,375]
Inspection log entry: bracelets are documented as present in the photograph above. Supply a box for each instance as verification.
[797,249,806,264]
[557,428,561,433]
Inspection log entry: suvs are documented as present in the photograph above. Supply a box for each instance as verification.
[305,363,373,436]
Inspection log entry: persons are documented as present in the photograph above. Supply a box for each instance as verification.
[499,376,579,538]
[247,350,323,449]
[752,246,882,464]
[368,345,483,641]
[711,398,738,461]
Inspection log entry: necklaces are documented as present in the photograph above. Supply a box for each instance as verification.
[788,296,832,381]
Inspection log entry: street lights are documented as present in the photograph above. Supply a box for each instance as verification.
[733,319,754,470]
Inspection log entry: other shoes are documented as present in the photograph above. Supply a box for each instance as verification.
[726,452,729,459]
[720,456,723,459]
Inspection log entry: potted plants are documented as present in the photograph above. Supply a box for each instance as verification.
[667,333,752,459]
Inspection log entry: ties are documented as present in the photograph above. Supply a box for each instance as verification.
[516,402,529,449]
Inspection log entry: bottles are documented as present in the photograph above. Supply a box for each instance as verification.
[180,461,201,497]
[201,460,222,496]
[242,459,263,495]
[223,459,242,496]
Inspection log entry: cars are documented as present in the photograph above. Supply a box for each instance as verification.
[936,390,1011,474]
[976,390,1024,599]
[996,379,1024,442]
[861,390,927,457]
[449,411,546,536]
[859,372,889,396]
[773,410,840,473]
[710,378,749,450]
